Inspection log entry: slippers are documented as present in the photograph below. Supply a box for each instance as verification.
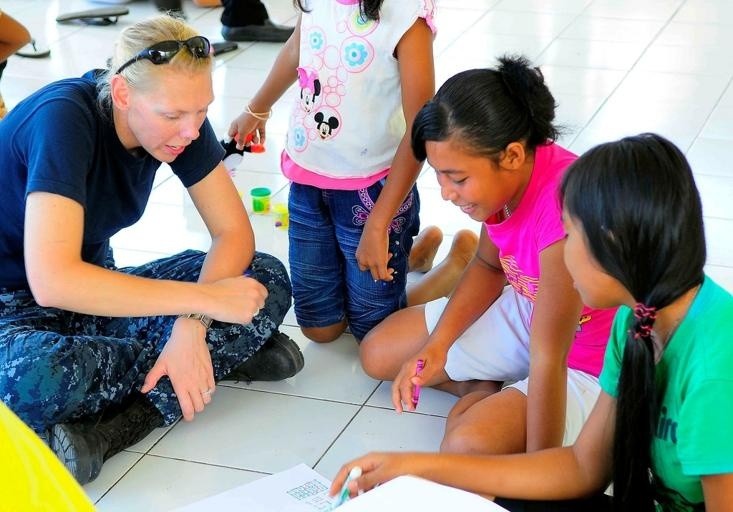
[56,7,128,24]
[14,38,50,57]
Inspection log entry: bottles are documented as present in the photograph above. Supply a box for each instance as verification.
[249,188,270,216]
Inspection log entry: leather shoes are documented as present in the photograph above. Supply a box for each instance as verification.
[222,19,295,42]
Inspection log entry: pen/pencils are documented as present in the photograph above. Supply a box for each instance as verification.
[335,466,362,508]
[413,360,424,409]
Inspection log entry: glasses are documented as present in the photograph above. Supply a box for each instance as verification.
[116,37,210,74]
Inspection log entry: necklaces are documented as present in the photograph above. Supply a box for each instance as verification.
[503,202,512,218]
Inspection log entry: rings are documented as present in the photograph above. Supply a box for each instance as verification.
[201,388,212,397]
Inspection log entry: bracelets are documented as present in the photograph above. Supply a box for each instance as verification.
[181,313,213,328]
[244,104,273,121]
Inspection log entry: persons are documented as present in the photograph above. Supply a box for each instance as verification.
[228,1,479,344]
[359,55,620,397]
[329,132,733,511]
[0,16,305,485]
[155,0,296,42]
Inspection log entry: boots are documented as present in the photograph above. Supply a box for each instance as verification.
[47,394,165,485]
[208,331,304,381]
